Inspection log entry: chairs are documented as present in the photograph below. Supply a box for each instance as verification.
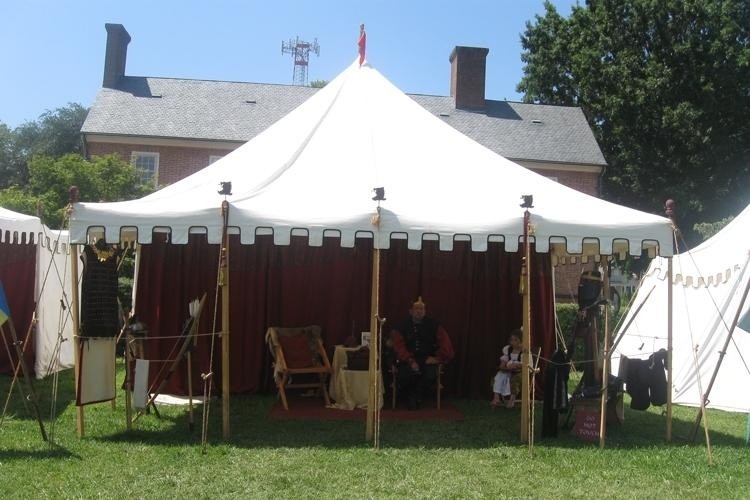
[267,325,335,410]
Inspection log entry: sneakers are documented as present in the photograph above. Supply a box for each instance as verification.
[407,397,425,410]
[489,399,515,409]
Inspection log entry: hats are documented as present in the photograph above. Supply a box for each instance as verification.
[411,296,426,305]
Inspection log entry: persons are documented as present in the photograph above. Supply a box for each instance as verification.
[489,329,536,408]
[391,294,455,412]
